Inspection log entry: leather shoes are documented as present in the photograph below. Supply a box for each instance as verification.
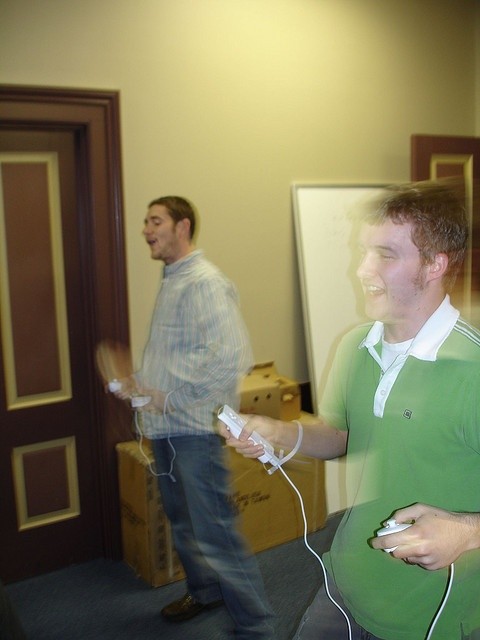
[161,588,225,622]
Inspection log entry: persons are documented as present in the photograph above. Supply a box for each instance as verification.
[102,195,282,640]
[213,180,478,640]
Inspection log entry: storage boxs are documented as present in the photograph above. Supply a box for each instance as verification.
[115,410,328,589]
[236,372,282,419]
